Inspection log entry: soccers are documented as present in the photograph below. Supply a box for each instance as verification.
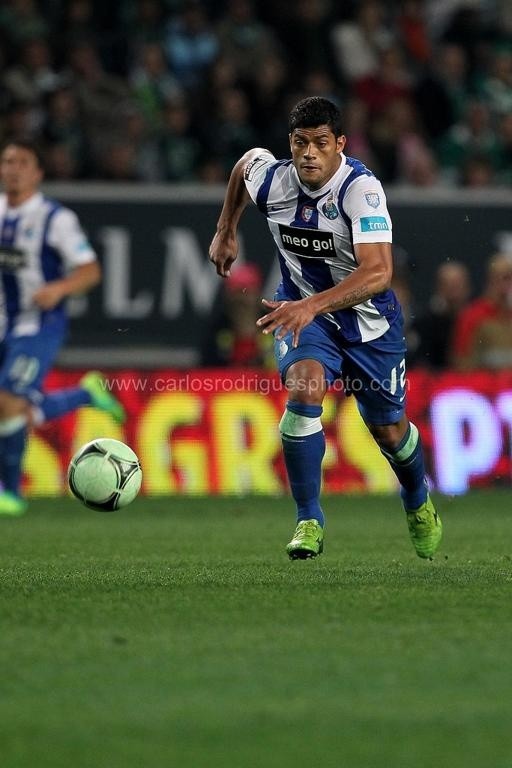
[68,438,142,512]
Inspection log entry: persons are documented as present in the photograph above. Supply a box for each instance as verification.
[0,140,103,515]
[0,0,512,370]
[208,94,444,559]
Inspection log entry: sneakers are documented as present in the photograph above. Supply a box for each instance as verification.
[286,518,324,558]
[83,369,125,423]
[1,490,27,516]
[406,491,442,557]
